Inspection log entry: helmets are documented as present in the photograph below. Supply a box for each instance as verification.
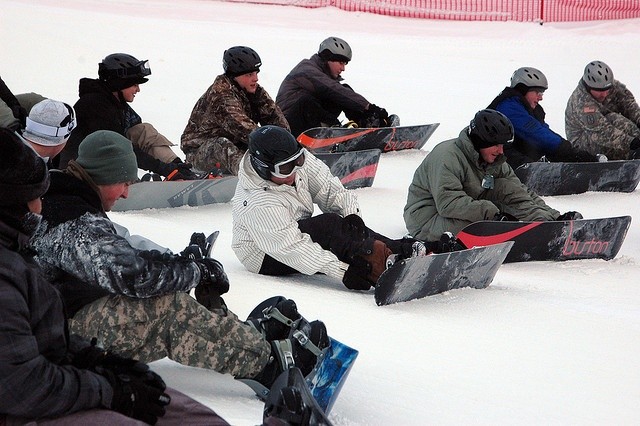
[468,109,515,149]
[98,53,151,92]
[222,46,262,77]
[319,37,352,60]
[511,67,548,89]
[22,99,77,146]
[248,125,306,180]
[583,61,613,90]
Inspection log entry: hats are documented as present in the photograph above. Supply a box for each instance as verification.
[76,130,138,184]
[1,126,50,202]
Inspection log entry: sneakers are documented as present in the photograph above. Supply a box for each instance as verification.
[391,242,426,258]
[243,299,297,341]
[254,320,328,395]
[379,114,400,126]
[430,231,455,253]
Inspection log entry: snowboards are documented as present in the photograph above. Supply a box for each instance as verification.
[191,231,220,258]
[375,240,517,306]
[247,296,359,416]
[314,149,381,189]
[453,215,633,263]
[263,369,333,426]
[109,176,239,210]
[298,122,440,152]
[514,160,635,195]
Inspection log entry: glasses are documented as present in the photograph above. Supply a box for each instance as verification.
[26,102,78,136]
[268,141,305,179]
[530,90,544,96]
[104,59,151,76]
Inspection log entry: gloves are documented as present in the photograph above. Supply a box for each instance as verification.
[193,258,229,296]
[342,267,372,291]
[157,160,192,179]
[368,102,388,119]
[95,349,171,425]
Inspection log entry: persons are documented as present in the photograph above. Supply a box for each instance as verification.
[0,127,305,426]
[275,37,400,140]
[45,53,223,182]
[28,130,327,403]
[403,109,583,243]
[15,99,207,260]
[231,125,455,291]
[486,67,608,170]
[565,60,640,160]
[0,75,48,135]
[181,46,291,176]
